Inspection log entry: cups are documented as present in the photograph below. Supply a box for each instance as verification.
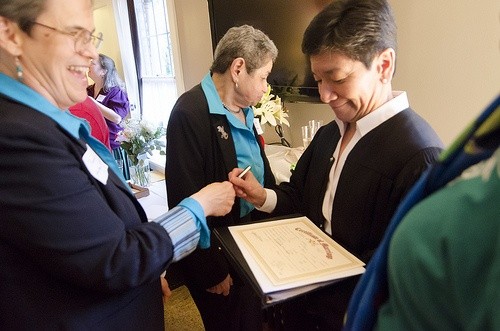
[114,159,123,177]
[301,120,321,150]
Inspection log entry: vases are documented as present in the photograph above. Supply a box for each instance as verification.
[131,156,148,188]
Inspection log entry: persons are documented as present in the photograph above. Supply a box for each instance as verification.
[229,0,444,331]
[344,149,500,331]
[0,0,236,331]
[67,54,131,178]
[164,25,278,331]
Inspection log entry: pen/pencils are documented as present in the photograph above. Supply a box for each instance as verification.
[232,166,251,184]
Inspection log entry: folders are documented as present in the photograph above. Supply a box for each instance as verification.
[212,213,352,309]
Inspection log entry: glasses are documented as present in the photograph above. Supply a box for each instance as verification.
[31,21,103,53]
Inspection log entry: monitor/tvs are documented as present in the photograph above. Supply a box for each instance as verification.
[205,0,334,104]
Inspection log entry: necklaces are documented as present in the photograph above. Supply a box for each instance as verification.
[222,102,241,113]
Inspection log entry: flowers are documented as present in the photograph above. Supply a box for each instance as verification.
[115,115,166,186]
[250,85,292,130]
